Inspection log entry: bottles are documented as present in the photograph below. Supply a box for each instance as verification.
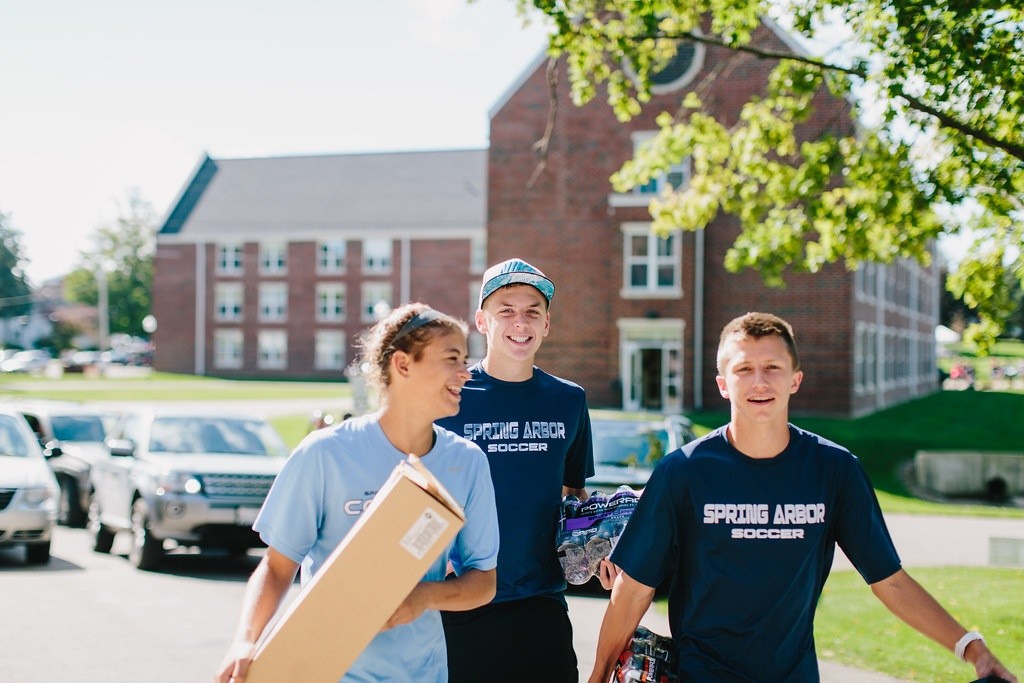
[554,484,646,586]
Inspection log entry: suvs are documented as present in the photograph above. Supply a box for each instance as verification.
[86,405,294,570]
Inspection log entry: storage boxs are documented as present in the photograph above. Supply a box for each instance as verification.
[244,453,468,683]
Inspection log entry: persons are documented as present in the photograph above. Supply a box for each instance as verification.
[588,312,1017,683]
[215,302,499,683]
[434,258,624,683]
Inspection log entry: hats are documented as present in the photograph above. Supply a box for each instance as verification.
[479,258,555,312]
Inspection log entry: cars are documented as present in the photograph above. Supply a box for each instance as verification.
[0,398,114,561]
[586,411,697,495]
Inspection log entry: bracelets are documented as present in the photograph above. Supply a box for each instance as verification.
[955,631,983,664]
